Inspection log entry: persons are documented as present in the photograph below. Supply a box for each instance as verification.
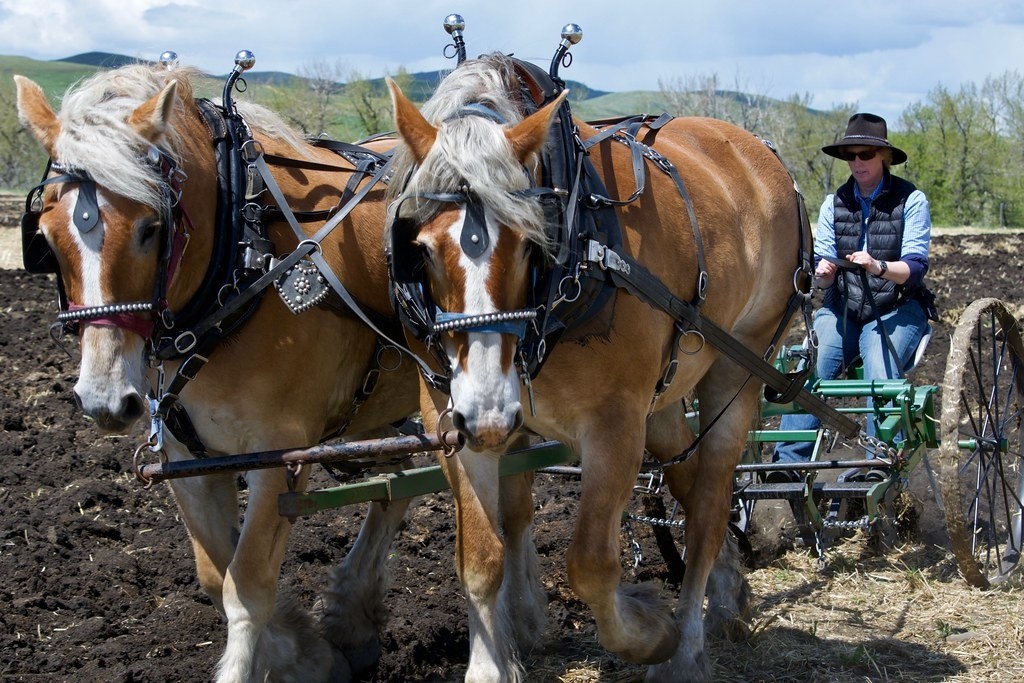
[765,111,931,484]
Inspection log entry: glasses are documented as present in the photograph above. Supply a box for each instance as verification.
[841,149,880,162]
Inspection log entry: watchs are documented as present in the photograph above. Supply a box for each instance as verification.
[870,259,887,277]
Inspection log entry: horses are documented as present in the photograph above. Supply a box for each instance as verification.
[14,52,815,683]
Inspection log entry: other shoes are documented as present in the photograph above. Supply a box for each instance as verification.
[766,472,792,483]
[865,470,888,481]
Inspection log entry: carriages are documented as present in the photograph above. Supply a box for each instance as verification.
[7,11,1024,683]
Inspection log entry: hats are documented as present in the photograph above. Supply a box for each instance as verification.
[822,113,907,165]
